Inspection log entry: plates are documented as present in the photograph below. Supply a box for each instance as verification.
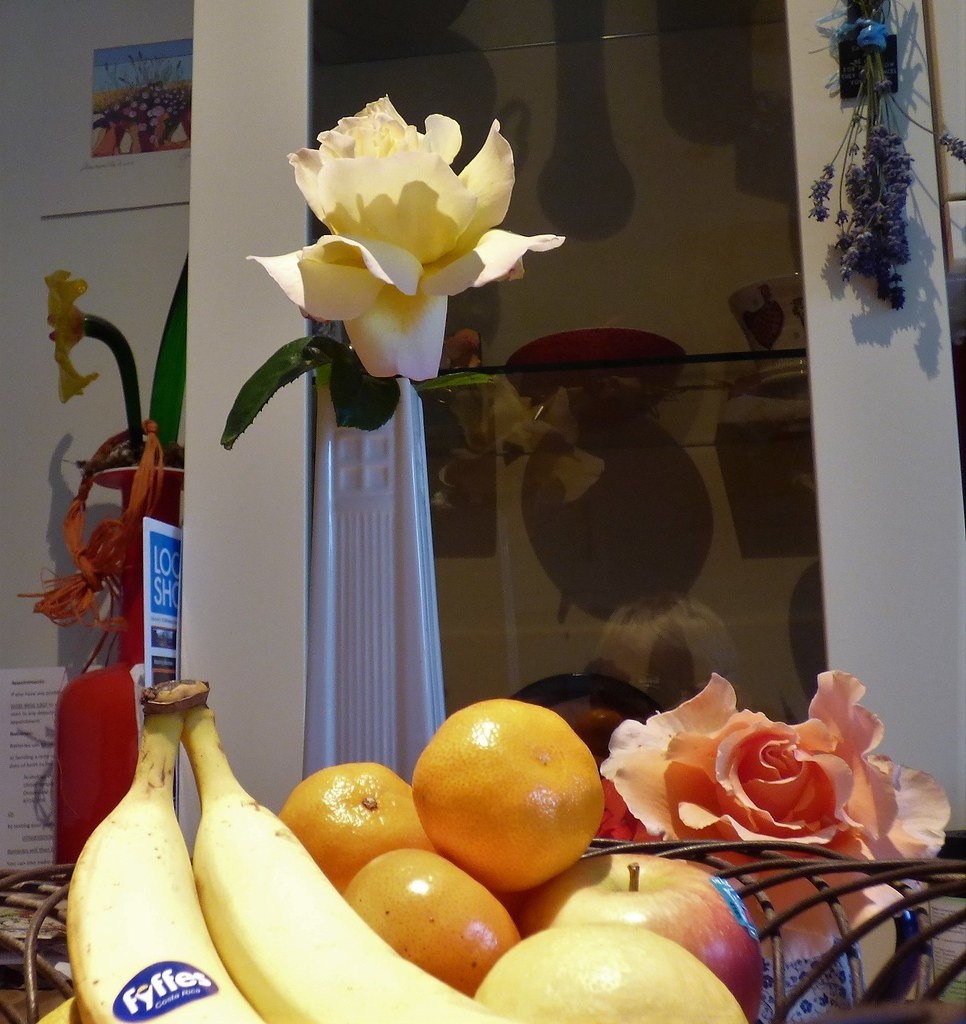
[511,674,662,781]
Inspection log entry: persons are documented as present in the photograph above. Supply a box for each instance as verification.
[591,595,734,716]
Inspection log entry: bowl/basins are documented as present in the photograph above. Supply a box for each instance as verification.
[506,328,686,426]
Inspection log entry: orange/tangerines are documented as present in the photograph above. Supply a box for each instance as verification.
[278,698,604,1000]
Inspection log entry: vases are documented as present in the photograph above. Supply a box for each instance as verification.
[304,384,448,787]
[49,465,186,870]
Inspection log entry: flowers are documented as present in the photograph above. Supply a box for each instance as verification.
[799,0,965,309]
[18,254,189,634]
[596,663,954,952]
[216,95,568,447]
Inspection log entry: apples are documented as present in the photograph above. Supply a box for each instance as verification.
[512,855,761,1019]
[475,924,749,1024]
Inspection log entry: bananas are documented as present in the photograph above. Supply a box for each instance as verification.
[62,680,516,1024]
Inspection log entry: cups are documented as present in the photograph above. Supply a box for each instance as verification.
[727,271,808,384]
